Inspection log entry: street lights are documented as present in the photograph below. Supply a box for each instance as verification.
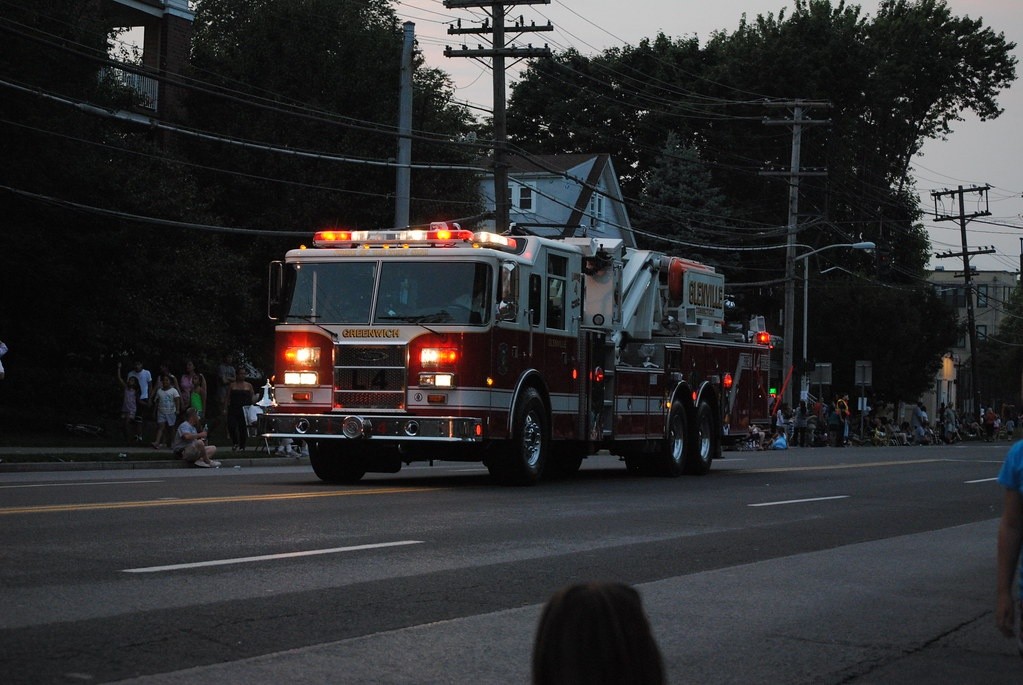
[780,240,876,432]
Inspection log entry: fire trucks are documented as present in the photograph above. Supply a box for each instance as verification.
[258,221,778,488]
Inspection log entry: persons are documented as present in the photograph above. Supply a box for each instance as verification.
[533,583,667,685]
[0,341,8,463]
[754,375,766,417]
[736,392,1023,450]
[446,275,500,324]
[998,441,1023,654]
[118,354,302,468]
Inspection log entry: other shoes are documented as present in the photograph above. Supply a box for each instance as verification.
[152,442,159,449]
[195,460,210,467]
[237,448,246,453]
[302,446,309,456]
[233,443,238,449]
[275,449,287,456]
[207,460,222,467]
[286,450,302,457]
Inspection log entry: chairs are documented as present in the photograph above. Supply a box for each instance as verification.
[242,405,270,456]
[884,428,901,447]
